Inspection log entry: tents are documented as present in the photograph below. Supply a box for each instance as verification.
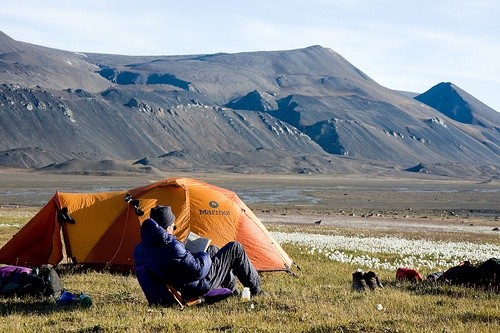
[0,177,302,277]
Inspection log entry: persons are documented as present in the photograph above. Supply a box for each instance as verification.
[133,205,270,308]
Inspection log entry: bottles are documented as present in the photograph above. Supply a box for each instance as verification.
[242,287,250,301]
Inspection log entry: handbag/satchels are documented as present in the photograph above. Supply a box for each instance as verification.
[36,264,64,296]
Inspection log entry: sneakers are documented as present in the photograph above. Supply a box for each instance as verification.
[252,287,270,298]
[227,285,242,296]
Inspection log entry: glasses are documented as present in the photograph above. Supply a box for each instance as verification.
[167,224,177,229]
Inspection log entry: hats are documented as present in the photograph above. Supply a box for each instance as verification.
[150,205,176,228]
